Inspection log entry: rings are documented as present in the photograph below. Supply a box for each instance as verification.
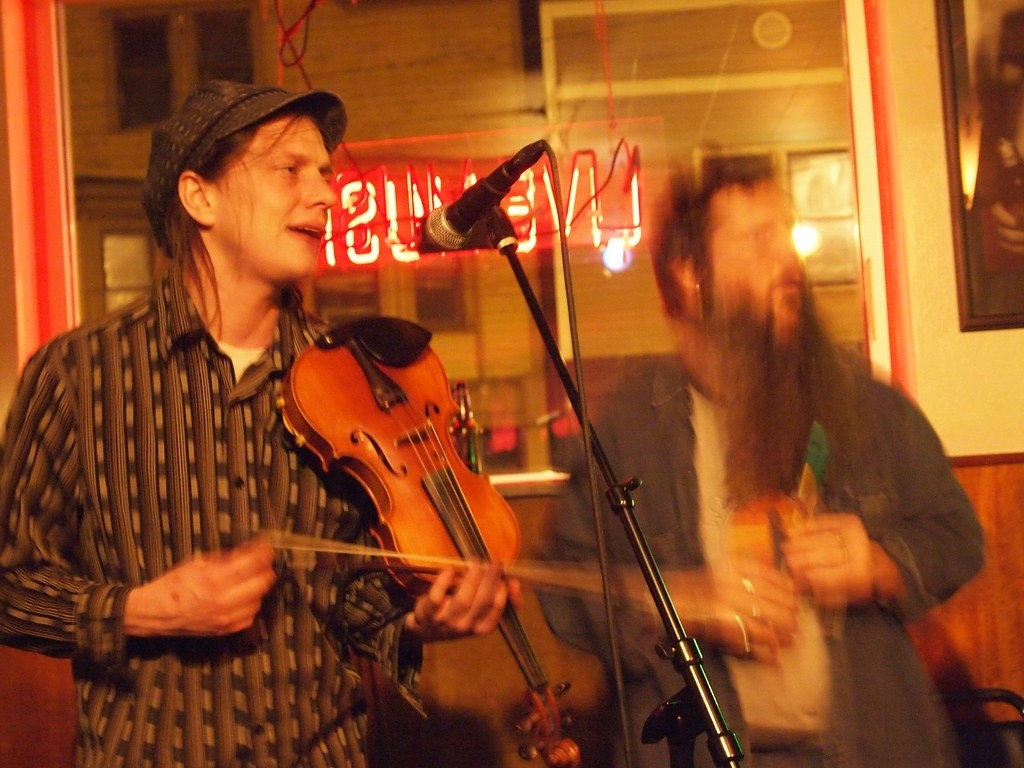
[834,531,852,561]
[741,576,756,594]
[750,602,767,620]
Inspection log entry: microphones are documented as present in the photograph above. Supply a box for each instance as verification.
[424,139,546,250]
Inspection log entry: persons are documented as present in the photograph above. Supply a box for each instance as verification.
[0,75,514,768]
[552,149,986,768]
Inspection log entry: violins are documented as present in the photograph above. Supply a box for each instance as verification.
[275,311,593,768]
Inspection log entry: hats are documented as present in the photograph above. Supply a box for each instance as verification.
[142,80,348,255]
